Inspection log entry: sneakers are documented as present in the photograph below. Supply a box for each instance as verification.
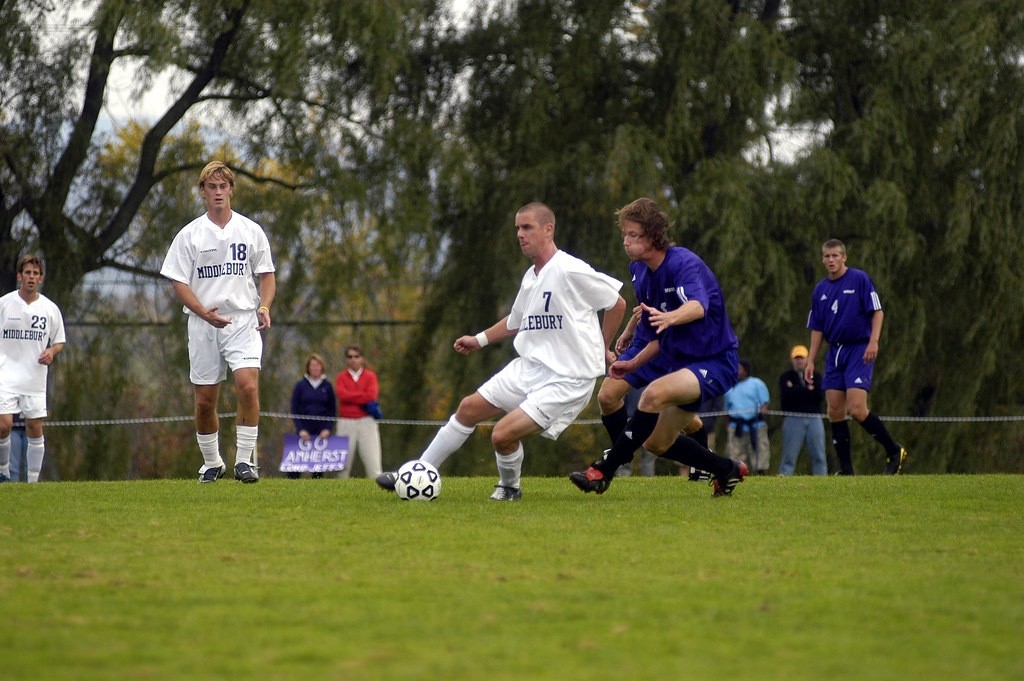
[708,460,749,497]
[375,470,398,493]
[490,485,523,502]
[198,463,226,483]
[832,470,854,476]
[569,460,612,495]
[884,442,908,475]
[233,463,260,484]
[688,467,711,482]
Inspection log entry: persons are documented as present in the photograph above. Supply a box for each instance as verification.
[804,239,907,477]
[568,198,739,496]
[375,203,627,504]
[159,161,277,484]
[722,360,771,476]
[334,345,385,480]
[289,354,338,479]
[0,256,67,483]
[598,304,749,498]
[776,344,828,476]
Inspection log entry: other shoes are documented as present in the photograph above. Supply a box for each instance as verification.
[0,473,10,483]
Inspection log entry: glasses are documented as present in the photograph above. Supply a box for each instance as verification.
[347,354,359,359]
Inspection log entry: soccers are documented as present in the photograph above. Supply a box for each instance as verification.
[395,460,442,502]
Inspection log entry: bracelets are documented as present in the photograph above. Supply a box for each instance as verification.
[475,331,489,348]
[257,307,269,313]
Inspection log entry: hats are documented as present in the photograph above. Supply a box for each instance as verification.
[791,345,809,358]
